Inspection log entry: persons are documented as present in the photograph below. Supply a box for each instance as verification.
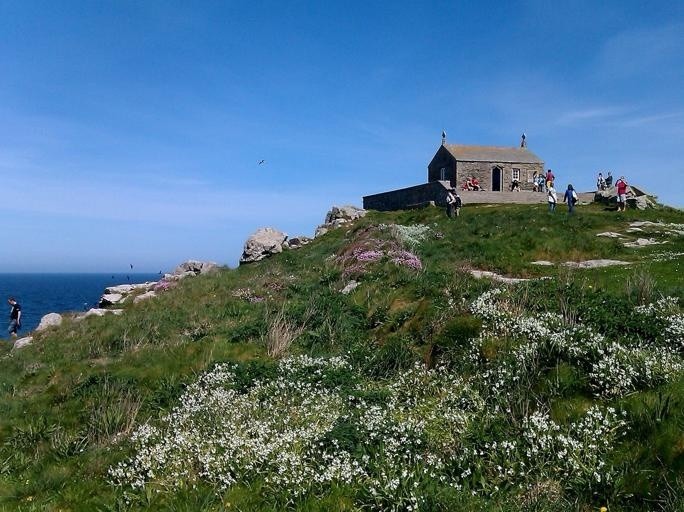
[445,175,484,218]
[84,301,88,310]
[7,296,21,344]
[512,169,629,212]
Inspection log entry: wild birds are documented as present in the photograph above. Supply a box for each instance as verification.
[158,270,163,275]
[129,263,134,270]
[259,159,265,165]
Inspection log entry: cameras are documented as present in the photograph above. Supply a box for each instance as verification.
[619,179,621,180]
